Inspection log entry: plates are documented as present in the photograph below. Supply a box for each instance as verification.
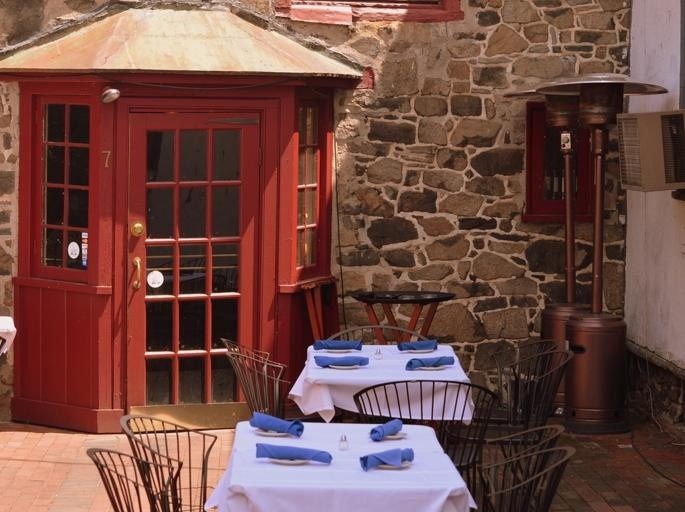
[323,348,354,354]
[330,364,361,371]
[267,458,311,466]
[405,349,434,355]
[417,366,449,373]
[384,432,408,440]
[379,463,412,470]
[254,429,290,437]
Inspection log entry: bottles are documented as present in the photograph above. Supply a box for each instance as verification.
[339,435,349,450]
[374,347,383,360]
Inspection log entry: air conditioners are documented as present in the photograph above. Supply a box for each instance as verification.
[615,109,685,193]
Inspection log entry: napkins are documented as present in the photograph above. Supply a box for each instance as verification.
[397,341,437,350]
[250,412,304,438]
[313,340,363,351]
[370,419,402,442]
[314,355,369,368]
[360,448,414,472]
[405,356,454,370]
[256,443,333,464]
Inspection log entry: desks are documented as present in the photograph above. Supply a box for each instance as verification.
[206,421,478,510]
[288,344,474,426]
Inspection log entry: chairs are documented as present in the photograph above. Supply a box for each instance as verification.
[473,340,563,476]
[221,337,286,416]
[87,448,174,511]
[120,413,218,511]
[444,350,575,499]
[327,326,428,347]
[353,379,501,498]
[485,425,561,508]
[482,446,578,509]
[227,350,316,421]
